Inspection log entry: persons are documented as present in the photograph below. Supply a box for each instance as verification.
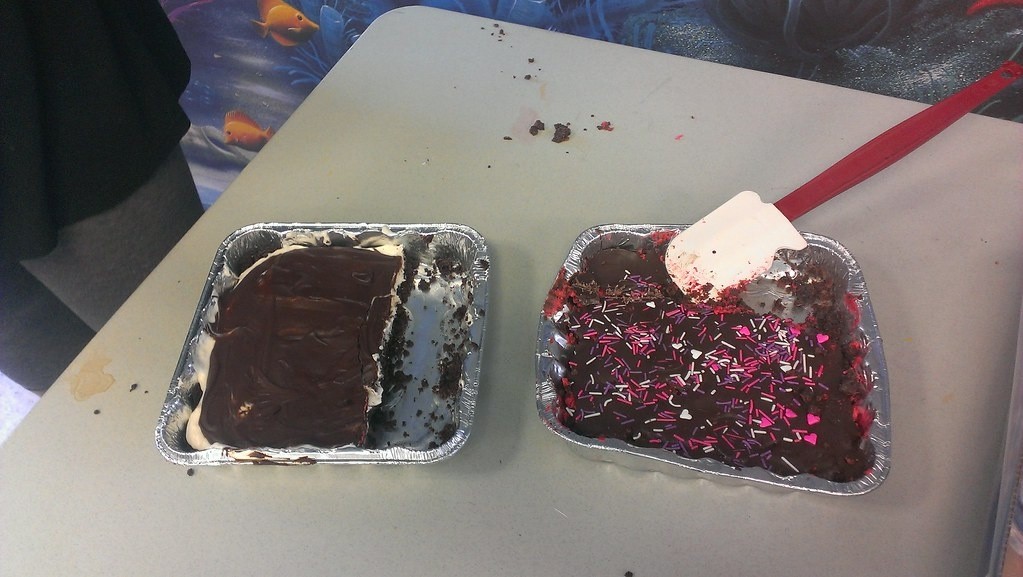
[0,0,204,399]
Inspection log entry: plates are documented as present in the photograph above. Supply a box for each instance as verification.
[533,223,893,497]
[153,220,492,467]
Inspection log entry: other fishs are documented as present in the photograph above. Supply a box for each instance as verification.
[223,111,274,152]
[966,0,1023,18]
[249,0,319,47]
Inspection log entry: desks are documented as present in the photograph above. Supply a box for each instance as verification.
[0,5,1023,577]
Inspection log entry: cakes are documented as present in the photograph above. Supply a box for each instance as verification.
[182,244,414,452]
[559,248,865,478]
